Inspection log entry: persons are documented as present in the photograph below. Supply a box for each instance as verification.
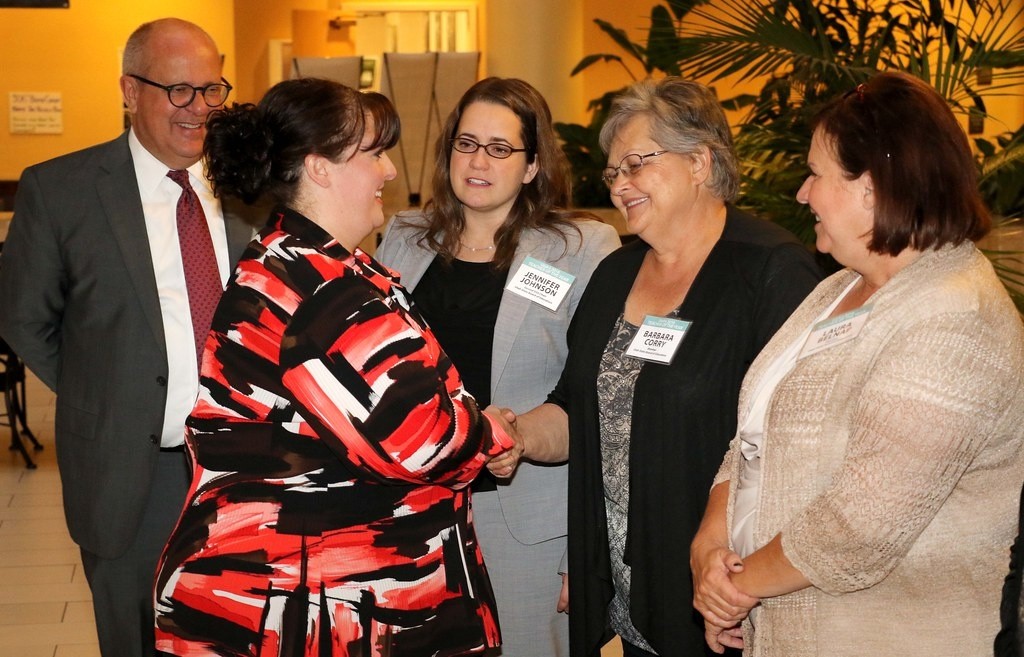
[487,76,822,657]
[0,19,258,657]
[690,69,1024,657]
[371,76,622,657]
[151,80,524,657]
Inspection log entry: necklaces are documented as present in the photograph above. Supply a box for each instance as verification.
[461,244,494,251]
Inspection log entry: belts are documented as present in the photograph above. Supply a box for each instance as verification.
[159,445,187,454]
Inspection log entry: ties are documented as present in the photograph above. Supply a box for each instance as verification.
[166,169,225,385]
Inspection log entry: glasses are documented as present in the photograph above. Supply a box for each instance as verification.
[449,135,529,160]
[125,75,233,107]
[843,82,892,158]
[601,150,668,188]
